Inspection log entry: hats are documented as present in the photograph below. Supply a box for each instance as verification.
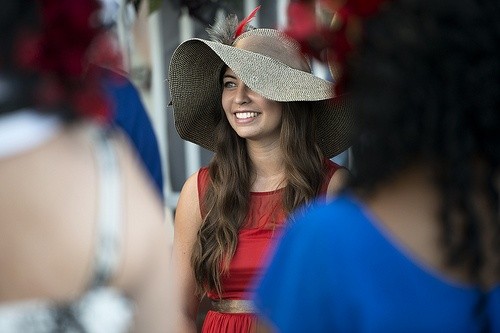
[168,4,353,159]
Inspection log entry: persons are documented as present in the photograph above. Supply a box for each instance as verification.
[247,0,500,333]
[168,5,360,333]
[0,0,198,333]
